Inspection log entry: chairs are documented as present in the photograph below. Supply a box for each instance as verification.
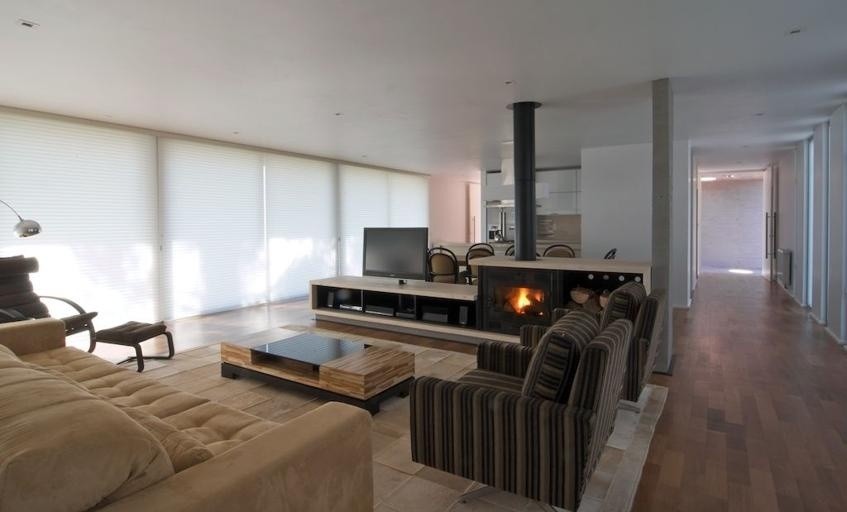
[426,243,617,287]
[409,311,633,512]
[0,255,98,354]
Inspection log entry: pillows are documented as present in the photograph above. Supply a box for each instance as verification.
[602,281,647,331]
[20,358,214,474]
[521,311,601,404]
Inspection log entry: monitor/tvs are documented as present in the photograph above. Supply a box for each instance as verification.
[362,226,430,285]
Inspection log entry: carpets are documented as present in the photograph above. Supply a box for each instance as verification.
[115,323,669,512]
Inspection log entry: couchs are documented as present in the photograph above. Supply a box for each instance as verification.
[0,318,374,512]
[521,282,665,402]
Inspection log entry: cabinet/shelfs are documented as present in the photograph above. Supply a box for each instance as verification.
[307,275,478,335]
[487,168,582,215]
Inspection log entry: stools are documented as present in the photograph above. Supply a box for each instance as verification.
[95,320,174,373]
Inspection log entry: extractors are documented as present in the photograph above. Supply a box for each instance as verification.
[482,158,550,201]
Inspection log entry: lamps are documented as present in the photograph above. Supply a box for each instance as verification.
[0,200,42,239]
